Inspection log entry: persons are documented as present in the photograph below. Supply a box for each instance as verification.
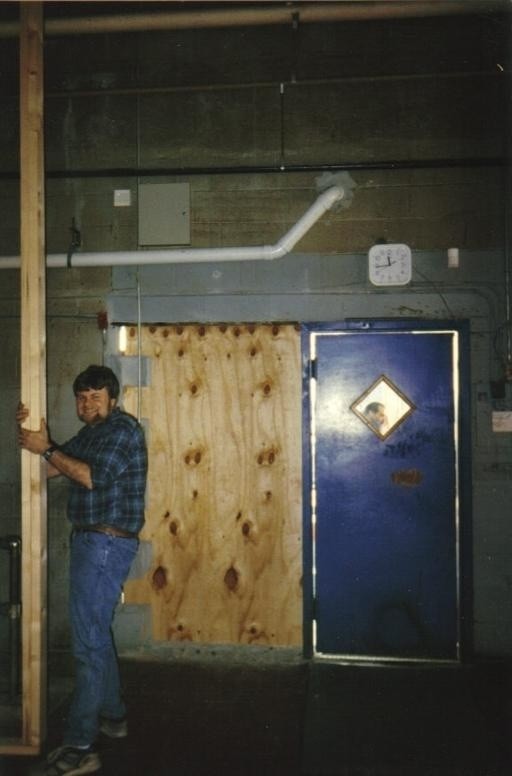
[366,402,389,434]
[14,365,148,775]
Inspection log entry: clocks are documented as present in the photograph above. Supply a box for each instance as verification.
[368,242,412,286]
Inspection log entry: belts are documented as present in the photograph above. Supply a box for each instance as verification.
[72,524,135,540]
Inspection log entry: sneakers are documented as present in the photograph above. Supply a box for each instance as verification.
[97,714,131,741]
[24,741,101,775]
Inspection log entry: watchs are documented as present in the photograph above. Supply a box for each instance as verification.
[43,446,57,461]
